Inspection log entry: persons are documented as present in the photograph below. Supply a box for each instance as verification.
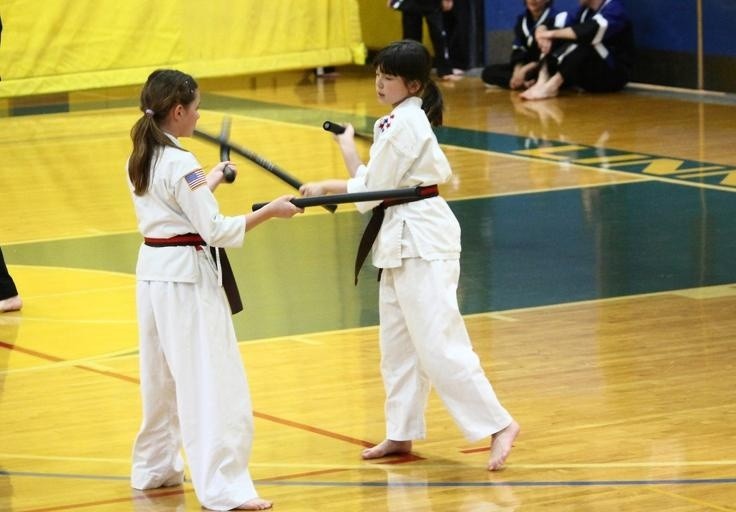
[299,40,522,472]
[1,251,22,312]
[131,72,304,512]
[314,66,341,78]
[389,0,461,81]
[519,0,636,100]
[481,0,573,90]
